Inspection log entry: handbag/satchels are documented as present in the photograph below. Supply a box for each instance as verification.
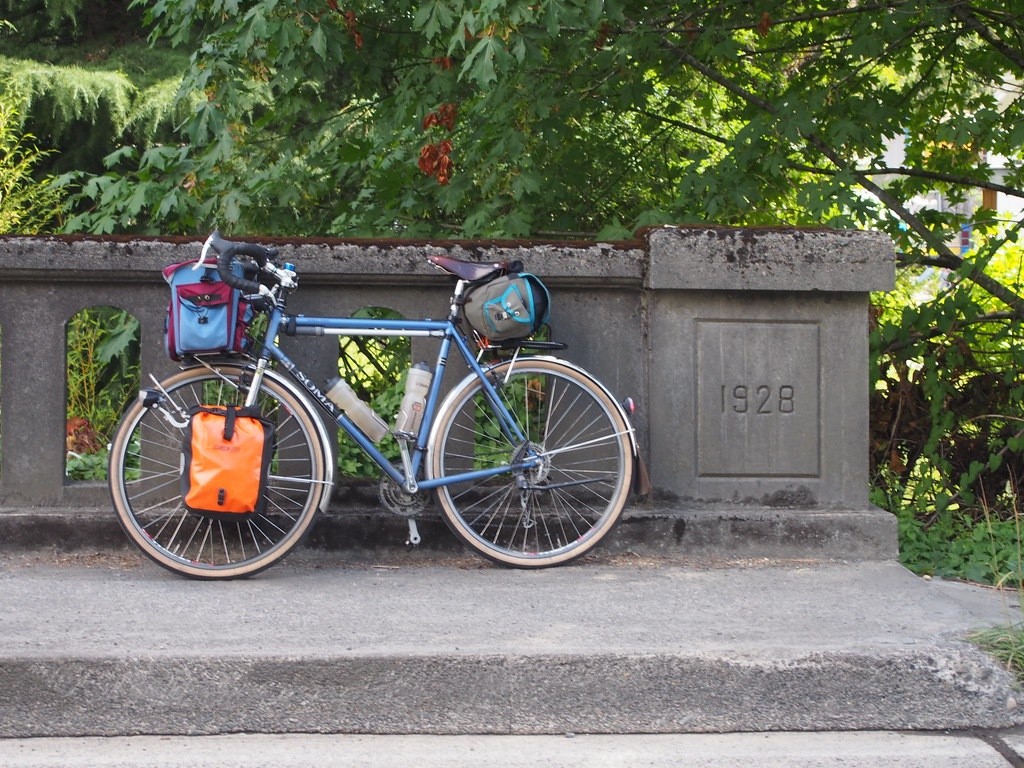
[463,268,553,341]
[178,404,278,521]
[161,258,258,363]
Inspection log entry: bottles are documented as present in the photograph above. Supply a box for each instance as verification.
[395,361,432,438]
[322,377,390,442]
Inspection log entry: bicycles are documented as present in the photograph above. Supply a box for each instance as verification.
[105,230,652,582]
